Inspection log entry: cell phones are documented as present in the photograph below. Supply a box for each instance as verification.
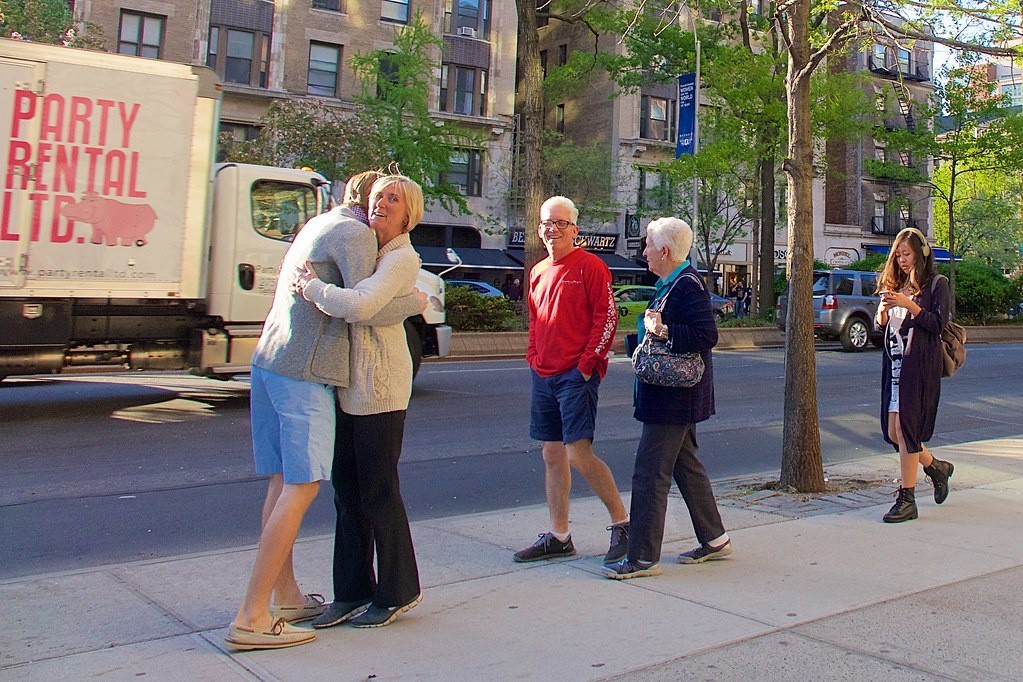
[879,290,892,299]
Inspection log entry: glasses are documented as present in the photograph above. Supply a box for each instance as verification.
[538,219,576,230]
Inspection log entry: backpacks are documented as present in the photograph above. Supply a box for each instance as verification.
[930,275,969,374]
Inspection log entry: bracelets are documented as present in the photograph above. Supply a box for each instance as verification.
[659,325,667,338]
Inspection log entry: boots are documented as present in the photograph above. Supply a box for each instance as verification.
[924,454,954,504]
[884,484,920,522]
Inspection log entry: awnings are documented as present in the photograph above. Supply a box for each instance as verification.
[414,246,522,269]
[867,245,963,260]
[504,248,647,270]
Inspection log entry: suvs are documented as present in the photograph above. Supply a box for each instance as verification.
[776,269,887,352]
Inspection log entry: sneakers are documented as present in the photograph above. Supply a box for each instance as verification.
[604,521,630,562]
[351,591,424,627]
[602,556,661,579]
[513,532,576,562]
[272,593,334,624]
[678,541,734,564]
[225,616,317,648]
[312,596,374,628]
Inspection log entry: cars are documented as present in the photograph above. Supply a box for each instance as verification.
[709,292,735,323]
[444,280,505,300]
[612,284,658,330]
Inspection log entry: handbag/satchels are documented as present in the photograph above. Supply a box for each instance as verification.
[632,272,706,386]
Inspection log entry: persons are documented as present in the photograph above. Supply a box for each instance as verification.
[601,216,733,579]
[223,171,428,650]
[501,277,520,301]
[871,227,954,525]
[620,291,633,302]
[513,195,630,564]
[732,281,752,319]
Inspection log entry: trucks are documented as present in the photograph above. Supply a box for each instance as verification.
[1,35,464,382]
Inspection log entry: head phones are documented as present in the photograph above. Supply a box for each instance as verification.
[896,227,930,256]
